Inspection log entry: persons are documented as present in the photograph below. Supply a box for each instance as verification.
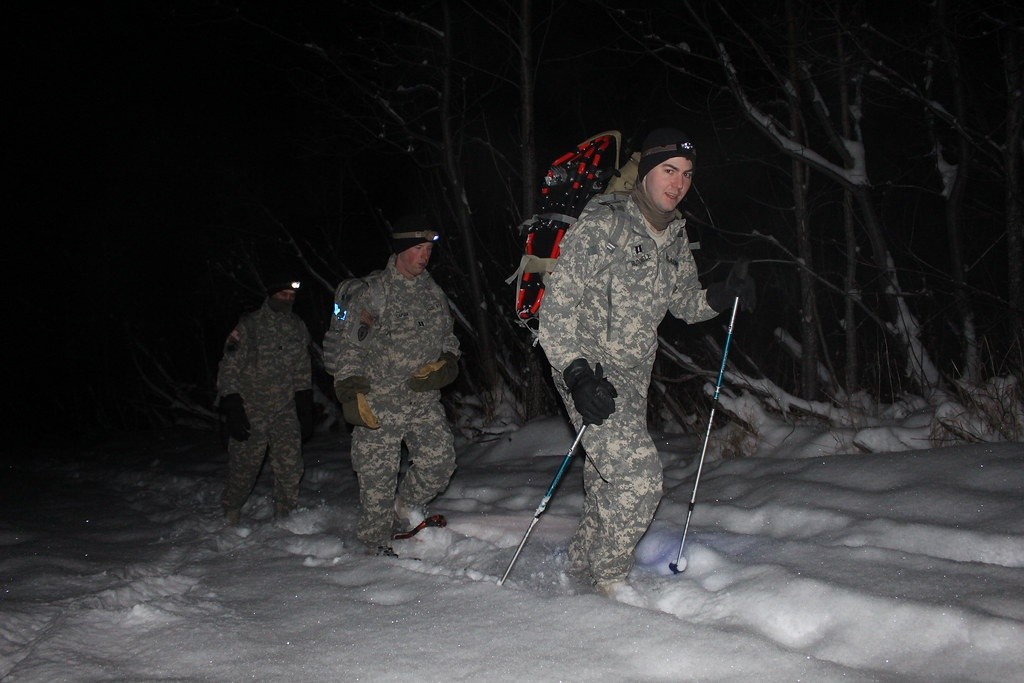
[217,270,315,526]
[537,128,754,601]
[322,213,462,554]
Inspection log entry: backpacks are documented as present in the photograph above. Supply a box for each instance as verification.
[505,131,629,340]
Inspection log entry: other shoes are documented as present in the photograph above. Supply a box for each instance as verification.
[367,544,398,557]
[393,493,424,534]
[592,577,629,597]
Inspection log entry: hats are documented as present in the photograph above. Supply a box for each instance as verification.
[269,272,298,295]
[392,216,433,254]
[638,126,696,182]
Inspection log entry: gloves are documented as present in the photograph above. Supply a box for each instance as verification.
[295,389,312,442]
[335,376,381,430]
[407,351,460,392]
[563,358,618,425]
[220,393,251,442]
[706,269,755,314]
